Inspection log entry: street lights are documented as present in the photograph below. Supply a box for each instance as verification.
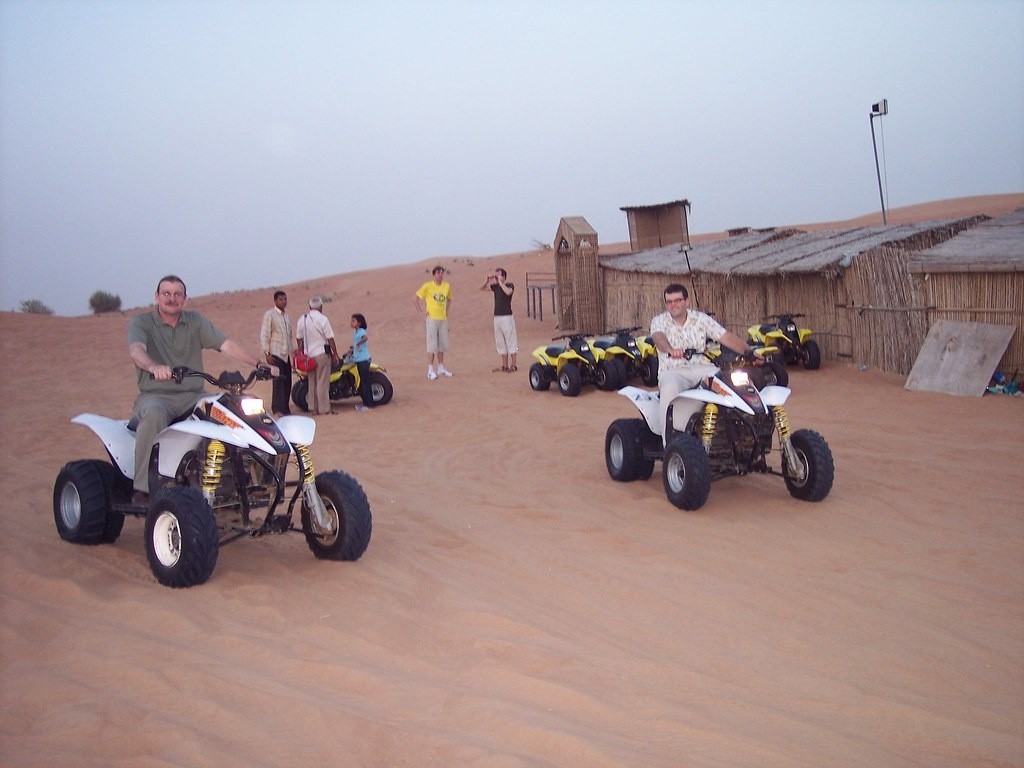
[869,98,888,226]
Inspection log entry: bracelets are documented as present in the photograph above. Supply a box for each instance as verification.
[256,360,262,369]
[265,352,270,356]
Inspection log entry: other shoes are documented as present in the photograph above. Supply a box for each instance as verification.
[427,371,438,380]
[437,369,453,377]
[492,365,509,372]
[131,491,151,509]
[320,410,339,415]
[355,403,373,410]
[274,412,284,418]
[313,412,320,415]
[507,365,518,373]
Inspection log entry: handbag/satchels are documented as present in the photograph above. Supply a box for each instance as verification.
[293,349,318,371]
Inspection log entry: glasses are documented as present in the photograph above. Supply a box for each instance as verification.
[665,299,684,304]
[158,291,185,298]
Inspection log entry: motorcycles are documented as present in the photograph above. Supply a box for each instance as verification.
[746,312,821,370]
[605,346,834,511]
[529,326,789,398]
[53,366,373,589]
[290,349,394,412]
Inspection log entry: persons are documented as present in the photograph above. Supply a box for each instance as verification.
[412,265,454,380]
[128,275,279,509]
[260,290,294,417]
[347,313,375,410]
[651,283,765,452]
[480,268,518,372]
[295,297,340,416]
